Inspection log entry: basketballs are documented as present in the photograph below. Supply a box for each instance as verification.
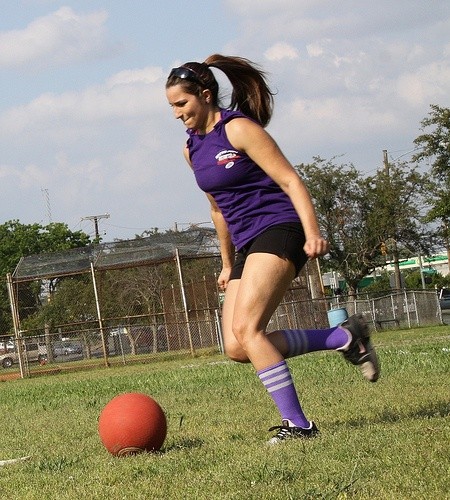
[98,392,168,459]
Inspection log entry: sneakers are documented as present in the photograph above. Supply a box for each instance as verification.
[334,312,379,382]
[266,419,319,445]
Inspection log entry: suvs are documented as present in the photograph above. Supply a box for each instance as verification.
[0,342,56,369]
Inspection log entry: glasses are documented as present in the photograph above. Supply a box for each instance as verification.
[167,67,213,99]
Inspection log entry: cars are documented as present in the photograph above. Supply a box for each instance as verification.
[362,288,450,318]
[53,343,82,356]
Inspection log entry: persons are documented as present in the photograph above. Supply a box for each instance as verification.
[165,55,382,448]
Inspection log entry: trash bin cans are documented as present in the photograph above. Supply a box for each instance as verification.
[327,306,348,329]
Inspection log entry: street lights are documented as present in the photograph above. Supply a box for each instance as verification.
[174,220,213,234]
[382,140,449,321]
